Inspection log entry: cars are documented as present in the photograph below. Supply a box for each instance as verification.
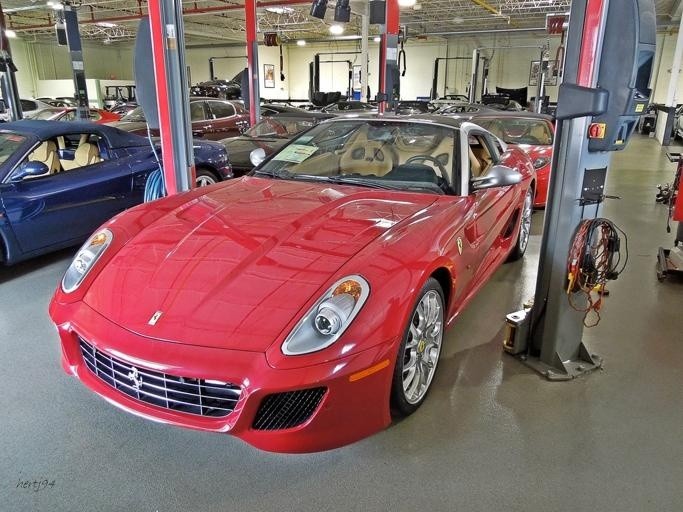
[673,105,682,140]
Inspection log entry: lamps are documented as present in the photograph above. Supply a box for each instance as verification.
[309,0,328,19]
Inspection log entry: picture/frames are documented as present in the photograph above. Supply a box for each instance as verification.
[529,60,557,86]
[264,64,275,88]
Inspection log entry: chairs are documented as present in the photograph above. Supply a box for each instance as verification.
[329,129,484,190]
[487,122,548,144]
[285,122,298,135]
[22,141,101,179]
[191,105,224,121]
[334,0,350,22]
